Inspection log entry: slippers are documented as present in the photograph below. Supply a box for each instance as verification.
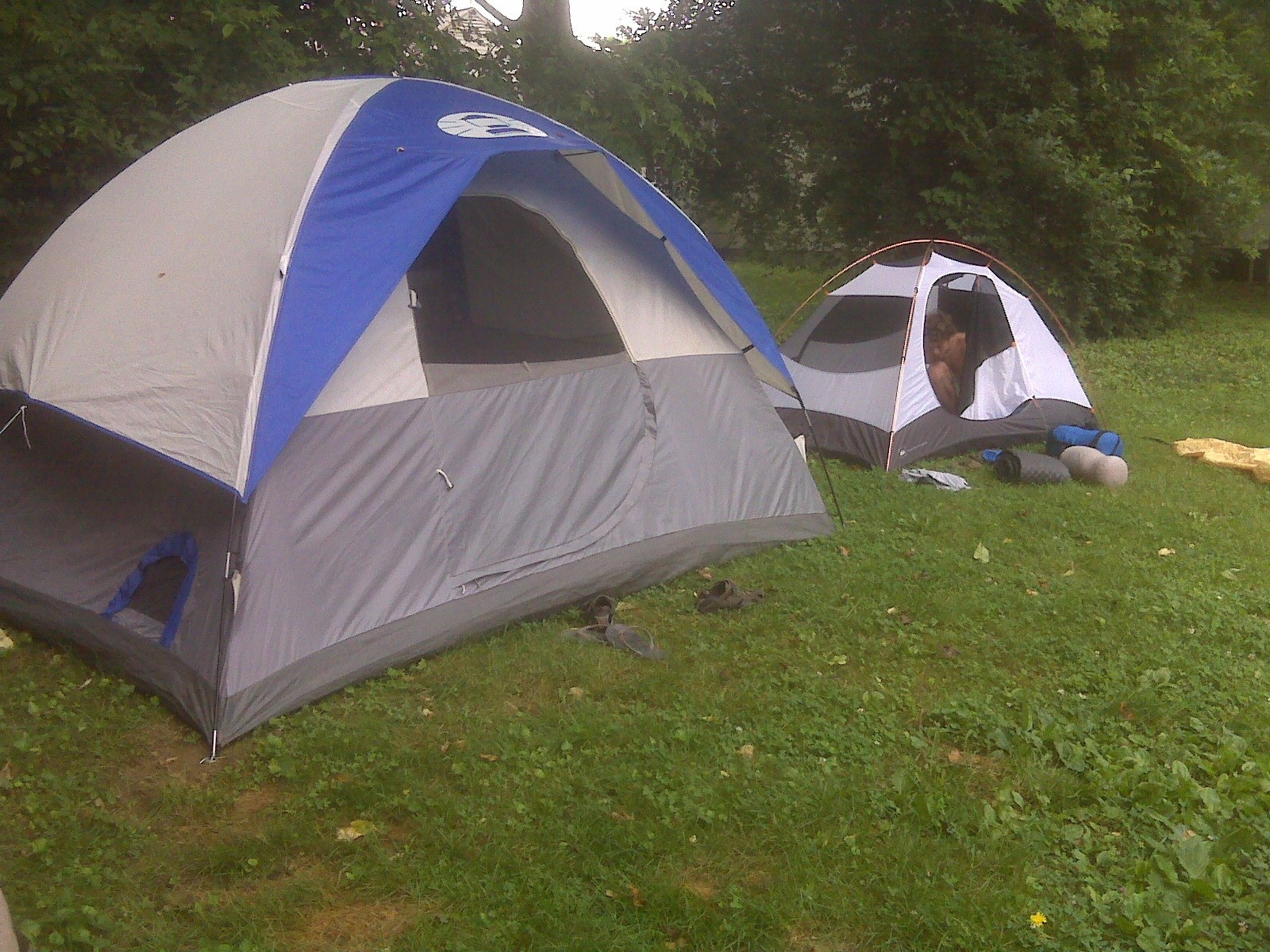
[560,625,608,645]
[605,624,667,661]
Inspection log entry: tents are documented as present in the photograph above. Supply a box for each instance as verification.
[1,73,846,765]
[777,240,1104,481]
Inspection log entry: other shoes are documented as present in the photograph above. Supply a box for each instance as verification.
[580,595,613,632]
[695,580,768,614]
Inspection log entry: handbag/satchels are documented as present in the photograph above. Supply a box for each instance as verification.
[1046,425,1122,459]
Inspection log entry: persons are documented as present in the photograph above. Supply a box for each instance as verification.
[921,310,991,418]
